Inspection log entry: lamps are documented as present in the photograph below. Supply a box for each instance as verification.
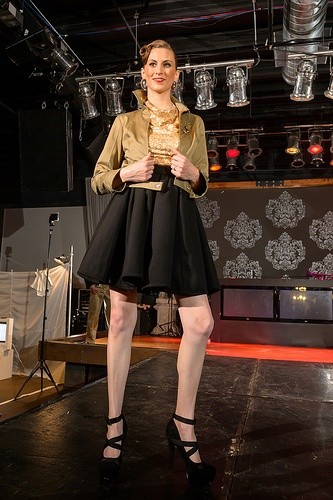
[0,0,333,119]
[206,126,333,171]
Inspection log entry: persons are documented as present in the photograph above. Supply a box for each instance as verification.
[78,40,224,482]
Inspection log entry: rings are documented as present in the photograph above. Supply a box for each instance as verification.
[172,165,176,171]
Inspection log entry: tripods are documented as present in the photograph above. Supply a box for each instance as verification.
[14,212,60,400]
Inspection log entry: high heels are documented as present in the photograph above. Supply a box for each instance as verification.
[166,413,217,485]
[100,415,127,483]
